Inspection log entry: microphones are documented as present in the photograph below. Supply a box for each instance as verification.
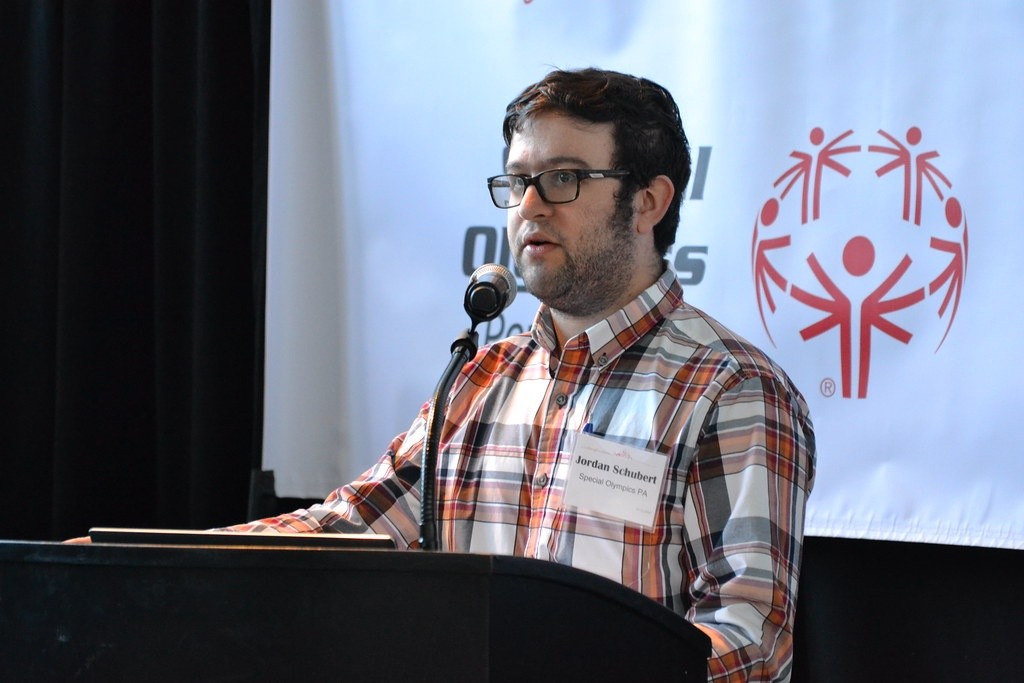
[465,262,517,314]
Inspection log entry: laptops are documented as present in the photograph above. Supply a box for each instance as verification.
[89,527,398,552]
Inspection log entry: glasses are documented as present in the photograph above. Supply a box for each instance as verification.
[487,168,632,209]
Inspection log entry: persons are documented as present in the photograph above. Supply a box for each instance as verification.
[62,66,816,683]
